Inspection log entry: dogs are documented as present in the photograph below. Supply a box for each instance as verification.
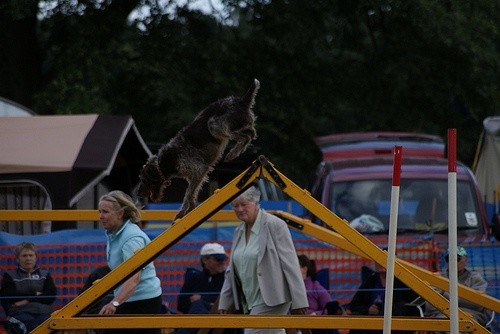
[134,76,260,223]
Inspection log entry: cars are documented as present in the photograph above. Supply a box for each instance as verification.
[316,131,491,270]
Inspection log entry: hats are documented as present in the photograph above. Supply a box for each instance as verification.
[199,243,228,262]
[444,246,468,257]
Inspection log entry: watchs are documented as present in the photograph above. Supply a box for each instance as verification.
[110,301,121,309]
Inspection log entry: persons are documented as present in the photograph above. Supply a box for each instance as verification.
[80,243,487,334]
[217,185,310,334]
[0,242,59,334]
[98,191,163,334]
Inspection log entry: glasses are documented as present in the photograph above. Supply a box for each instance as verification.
[445,256,462,263]
